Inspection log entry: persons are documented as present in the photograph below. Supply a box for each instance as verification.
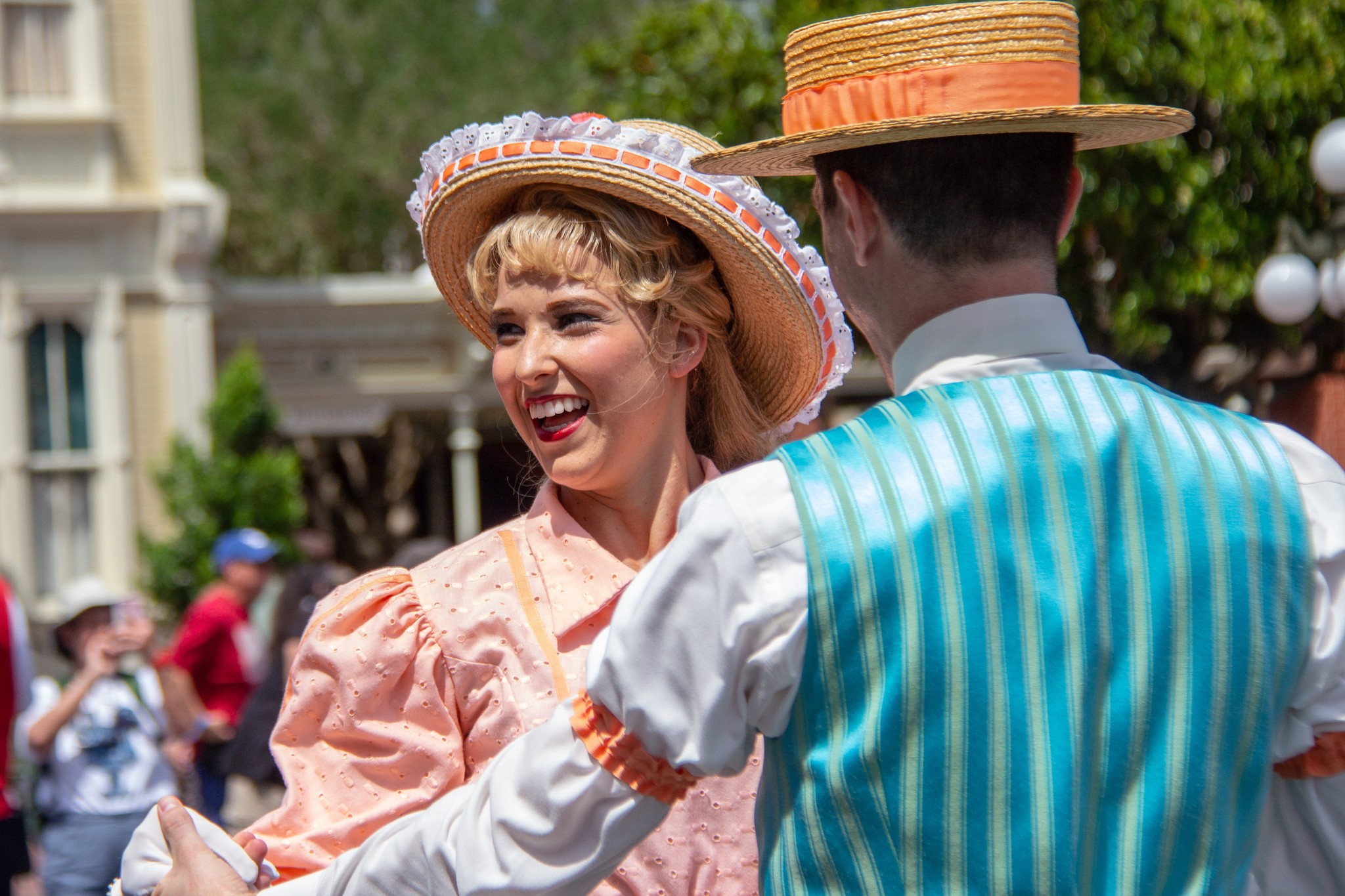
[108,112,855,896]
[150,0,1345,896]
[0,525,355,896]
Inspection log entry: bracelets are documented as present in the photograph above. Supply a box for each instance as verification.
[188,716,211,736]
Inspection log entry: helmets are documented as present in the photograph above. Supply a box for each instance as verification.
[33,578,132,659]
[211,526,284,572]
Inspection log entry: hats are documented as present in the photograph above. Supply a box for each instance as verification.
[404,111,854,439]
[689,2,1196,178]
